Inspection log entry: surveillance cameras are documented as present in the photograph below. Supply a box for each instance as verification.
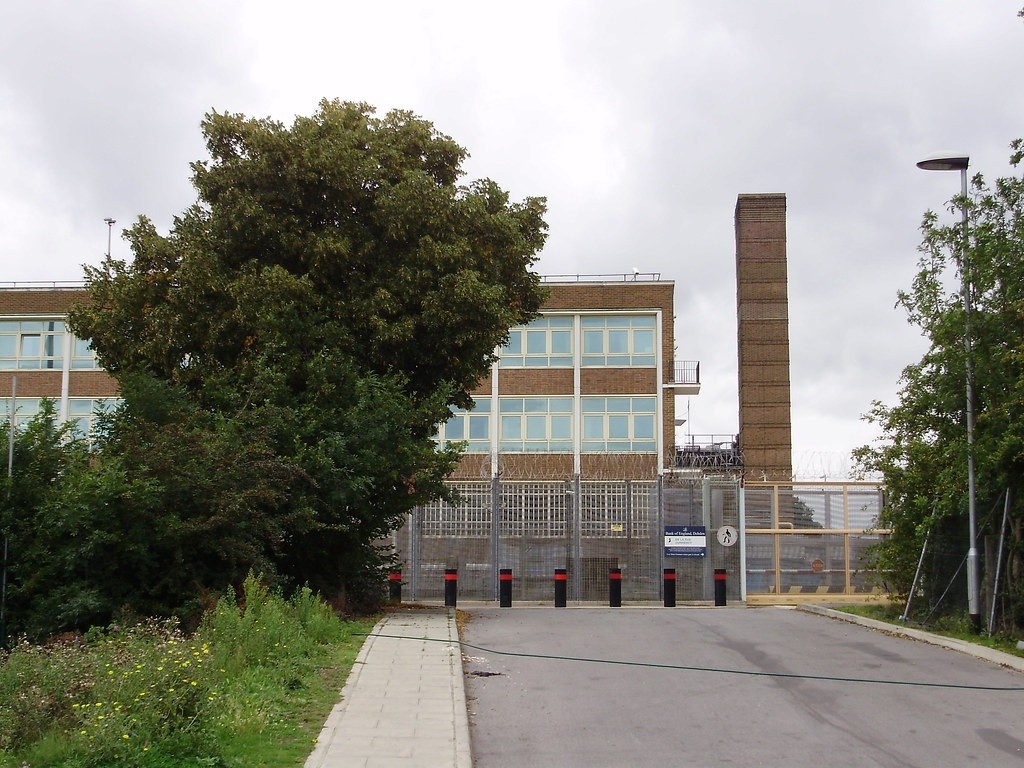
[104,217,116,224]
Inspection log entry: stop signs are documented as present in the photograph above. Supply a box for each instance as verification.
[811,559,824,571]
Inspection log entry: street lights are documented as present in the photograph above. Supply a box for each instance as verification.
[915,151,982,638]
